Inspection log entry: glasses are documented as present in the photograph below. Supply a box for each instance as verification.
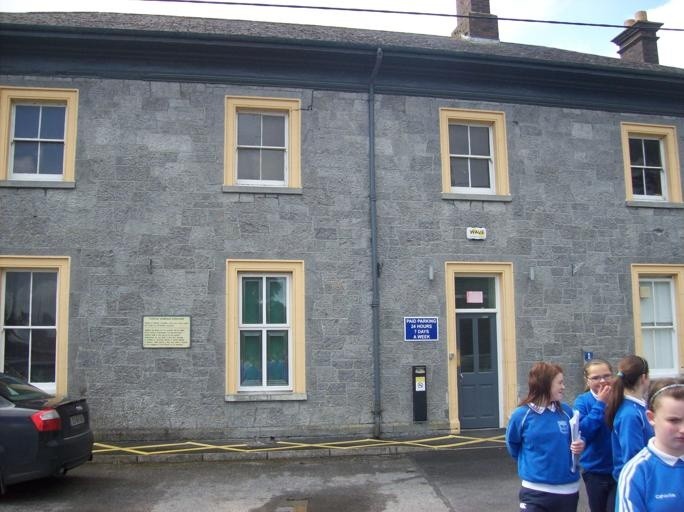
[586,375,614,382]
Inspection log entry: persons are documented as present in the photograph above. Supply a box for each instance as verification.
[504,354,684,512]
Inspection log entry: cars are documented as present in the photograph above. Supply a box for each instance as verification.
[0,372,94,491]
